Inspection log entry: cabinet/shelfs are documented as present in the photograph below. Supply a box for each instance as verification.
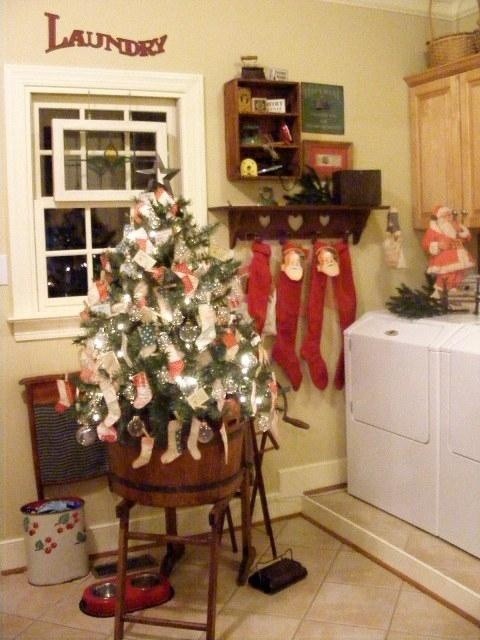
[403,66,480,231]
[224,77,304,182]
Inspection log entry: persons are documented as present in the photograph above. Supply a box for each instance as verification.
[420,203,475,305]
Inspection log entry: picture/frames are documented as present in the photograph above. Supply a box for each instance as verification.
[302,138,353,182]
[49,117,168,203]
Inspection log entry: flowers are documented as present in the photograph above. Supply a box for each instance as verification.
[283,166,333,205]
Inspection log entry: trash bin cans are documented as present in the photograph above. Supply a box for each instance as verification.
[20,496,91,586]
